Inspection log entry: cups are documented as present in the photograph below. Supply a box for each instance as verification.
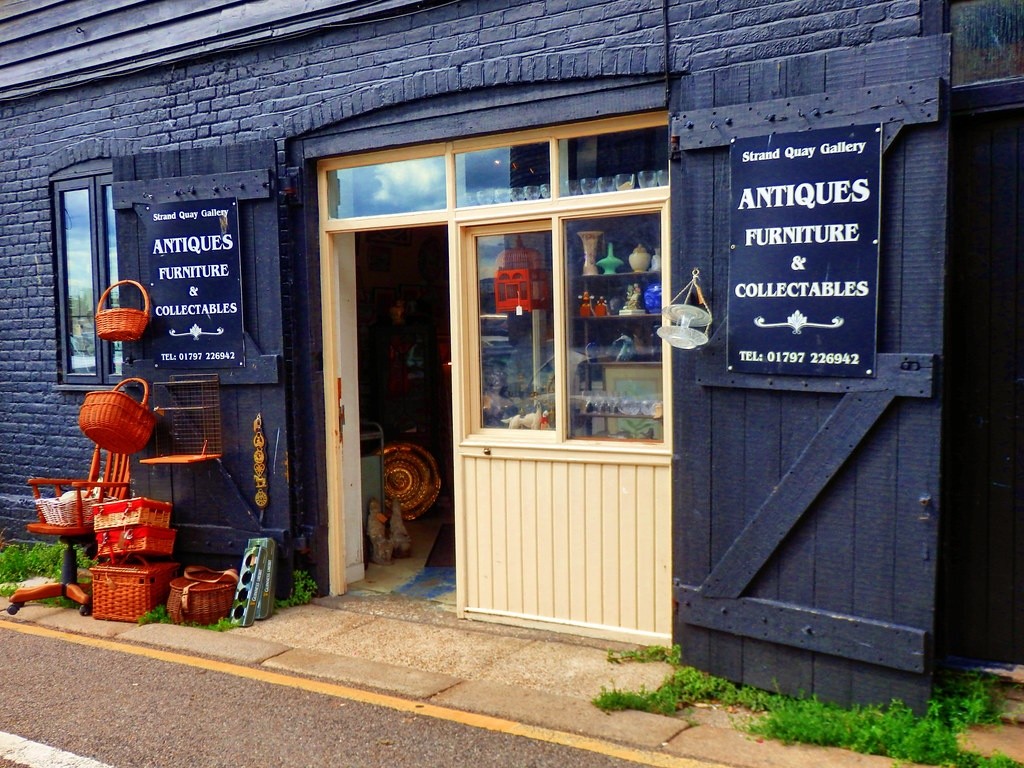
[477,190,494,205]
[579,389,663,417]
[508,187,525,202]
[494,188,511,203]
[598,177,615,192]
[637,171,657,188]
[580,178,597,194]
[656,169,669,186]
[568,180,582,195]
[523,186,541,200]
[540,184,550,198]
[615,173,635,191]
[462,192,477,206]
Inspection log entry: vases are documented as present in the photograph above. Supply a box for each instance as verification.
[577,231,603,276]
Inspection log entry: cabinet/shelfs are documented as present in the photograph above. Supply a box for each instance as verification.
[570,270,663,422]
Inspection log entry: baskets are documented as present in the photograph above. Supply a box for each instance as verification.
[95,280,151,342]
[90,497,174,531]
[89,555,180,623]
[79,378,158,455]
[95,526,176,554]
[166,572,238,626]
[36,496,118,527]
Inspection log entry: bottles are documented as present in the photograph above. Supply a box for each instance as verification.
[651,248,660,271]
[643,283,662,313]
[628,244,651,272]
[577,231,603,275]
[595,243,625,275]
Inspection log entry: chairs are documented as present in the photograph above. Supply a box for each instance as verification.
[7,442,131,615]
